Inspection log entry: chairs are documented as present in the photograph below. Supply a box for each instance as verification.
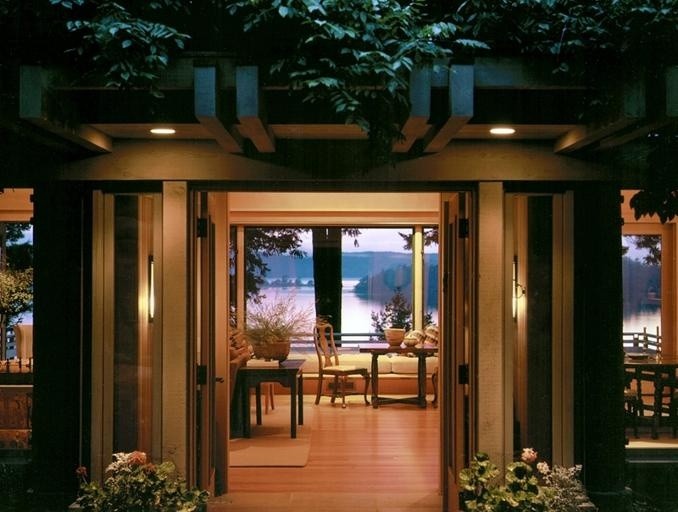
[623,391,641,444]
[431,368,440,408]
[313,323,371,408]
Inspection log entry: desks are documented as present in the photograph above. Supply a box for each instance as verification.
[238,359,306,438]
[624,346,678,439]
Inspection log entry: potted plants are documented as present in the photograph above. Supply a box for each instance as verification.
[228,288,331,363]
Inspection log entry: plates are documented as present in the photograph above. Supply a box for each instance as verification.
[626,352,650,359]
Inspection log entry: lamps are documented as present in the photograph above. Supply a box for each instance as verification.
[146,254,155,322]
[511,261,526,321]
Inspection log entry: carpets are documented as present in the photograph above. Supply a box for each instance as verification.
[228,405,314,467]
